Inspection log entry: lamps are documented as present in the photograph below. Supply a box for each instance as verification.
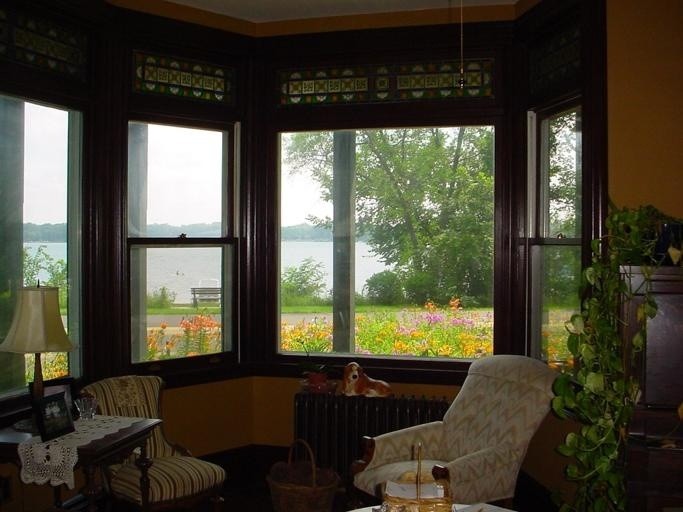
[1,279,74,439]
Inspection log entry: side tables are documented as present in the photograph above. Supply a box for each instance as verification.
[342,500,517,512]
[0,414,163,510]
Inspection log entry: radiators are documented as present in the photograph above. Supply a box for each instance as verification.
[293,391,447,479]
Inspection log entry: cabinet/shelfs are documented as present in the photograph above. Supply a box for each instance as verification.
[617,264,683,512]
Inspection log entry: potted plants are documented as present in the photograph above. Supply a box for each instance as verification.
[551,205,683,511]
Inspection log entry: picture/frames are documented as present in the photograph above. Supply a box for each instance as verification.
[32,390,76,441]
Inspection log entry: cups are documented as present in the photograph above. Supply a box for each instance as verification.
[73,397,100,423]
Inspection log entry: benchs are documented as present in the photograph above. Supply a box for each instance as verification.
[190,287,222,308]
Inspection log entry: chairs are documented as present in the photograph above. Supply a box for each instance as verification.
[347,355,563,506]
[79,375,226,510]
